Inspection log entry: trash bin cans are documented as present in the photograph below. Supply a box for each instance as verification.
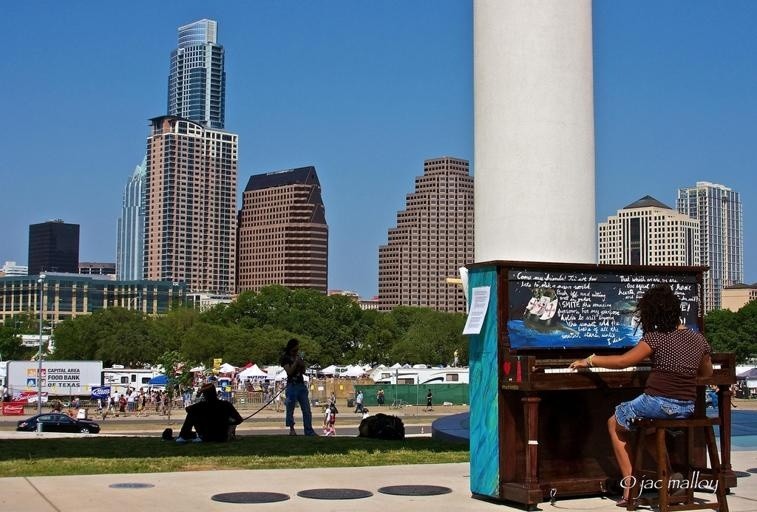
[37,423,43,432]
[348,399,353,407]
[128,402,135,410]
[96,399,102,408]
[240,397,245,404]
[311,400,318,406]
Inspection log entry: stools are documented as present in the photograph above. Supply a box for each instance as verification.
[623,414,731,512]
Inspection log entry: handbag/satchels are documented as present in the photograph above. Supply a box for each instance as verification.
[124,399,128,405]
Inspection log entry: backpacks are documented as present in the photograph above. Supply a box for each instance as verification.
[358,413,406,440]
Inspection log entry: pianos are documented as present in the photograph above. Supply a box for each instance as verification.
[464,261,738,510]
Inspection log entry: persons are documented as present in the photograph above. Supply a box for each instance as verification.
[51,338,386,444]
[568,283,713,508]
[708,376,757,408]
[423,389,433,411]
[451,348,459,367]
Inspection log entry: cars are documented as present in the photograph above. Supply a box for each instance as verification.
[15,414,103,436]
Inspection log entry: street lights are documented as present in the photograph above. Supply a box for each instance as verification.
[127,295,141,309]
[51,298,63,332]
[37,273,46,436]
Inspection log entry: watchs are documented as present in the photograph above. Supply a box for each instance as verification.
[586,352,596,367]
